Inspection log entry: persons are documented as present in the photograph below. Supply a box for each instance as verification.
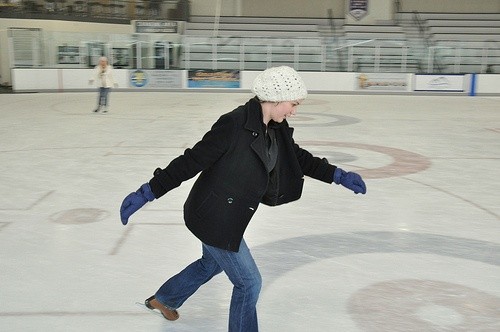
[88,56,119,113]
[119,64,367,331]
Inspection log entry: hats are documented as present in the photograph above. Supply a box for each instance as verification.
[252,66,308,102]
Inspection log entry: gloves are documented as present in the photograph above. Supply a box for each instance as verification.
[120,182,155,225]
[333,168,366,194]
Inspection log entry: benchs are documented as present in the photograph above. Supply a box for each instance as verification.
[179,13,500,73]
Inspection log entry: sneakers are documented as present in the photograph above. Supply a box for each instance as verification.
[136,294,180,322]
